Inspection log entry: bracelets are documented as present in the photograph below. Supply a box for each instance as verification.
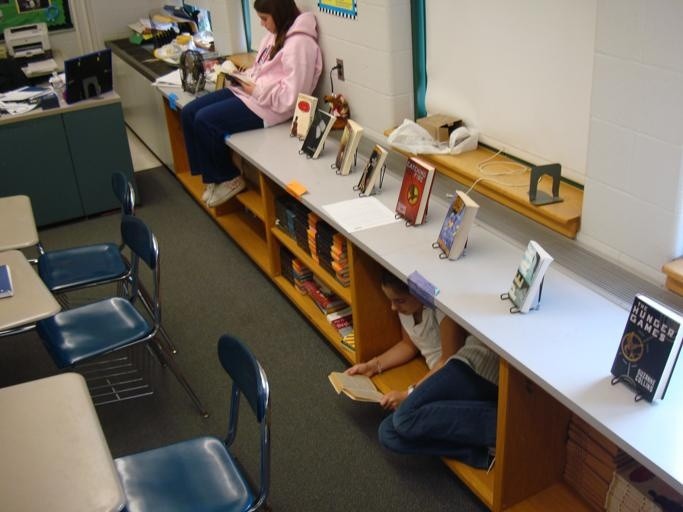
[373,357,382,374]
[407,383,414,395]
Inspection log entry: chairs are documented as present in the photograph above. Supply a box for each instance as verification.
[113,331,272,511]
[38,168,179,355]
[35,214,209,419]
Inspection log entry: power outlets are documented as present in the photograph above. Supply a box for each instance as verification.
[335,59,344,81]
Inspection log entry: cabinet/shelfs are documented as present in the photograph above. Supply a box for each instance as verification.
[158,79,683,511]
[105,34,219,174]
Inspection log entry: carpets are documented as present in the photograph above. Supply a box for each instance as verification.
[0,166,492,511]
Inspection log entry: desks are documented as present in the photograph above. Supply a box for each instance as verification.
[0,193,48,255]
[1,372,128,511]
[0,67,134,228]
[0,247,61,341]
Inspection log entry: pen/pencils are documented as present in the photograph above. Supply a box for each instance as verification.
[159,81,178,85]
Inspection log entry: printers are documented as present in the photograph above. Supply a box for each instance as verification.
[3,22,51,60]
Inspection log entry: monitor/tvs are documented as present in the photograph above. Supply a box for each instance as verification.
[63,48,114,104]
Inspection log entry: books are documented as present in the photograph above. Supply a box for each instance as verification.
[438,188,479,261]
[291,93,319,140]
[612,292,683,401]
[328,372,385,403]
[301,110,337,158]
[0,263,14,299]
[27,58,60,78]
[562,407,683,511]
[274,186,355,351]
[396,156,437,226]
[213,61,254,87]
[509,240,554,312]
[337,118,364,175]
[358,144,389,197]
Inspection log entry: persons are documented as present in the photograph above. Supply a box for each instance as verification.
[179,0,323,208]
[344,269,499,473]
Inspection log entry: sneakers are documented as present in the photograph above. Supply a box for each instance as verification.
[202,177,245,208]
